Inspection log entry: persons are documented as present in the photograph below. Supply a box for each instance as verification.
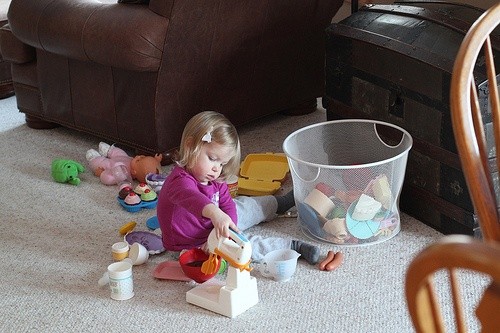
[86,142,162,185]
[157,111,320,264]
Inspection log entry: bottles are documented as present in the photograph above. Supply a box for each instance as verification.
[112,242,129,261]
[111,160,132,185]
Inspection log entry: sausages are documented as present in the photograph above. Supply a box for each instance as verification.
[320,250,344,270]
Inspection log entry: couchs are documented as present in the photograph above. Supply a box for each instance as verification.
[0,0,347,163]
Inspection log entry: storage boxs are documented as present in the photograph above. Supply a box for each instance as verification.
[237,152,291,197]
[323,0,500,241]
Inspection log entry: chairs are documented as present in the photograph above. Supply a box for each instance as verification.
[382,2,500,333]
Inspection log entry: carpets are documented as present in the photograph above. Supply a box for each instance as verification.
[0,98,493,333]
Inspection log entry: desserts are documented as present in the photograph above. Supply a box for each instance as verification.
[118,183,158,205]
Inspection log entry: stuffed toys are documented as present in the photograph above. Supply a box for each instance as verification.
[51,158,86,185]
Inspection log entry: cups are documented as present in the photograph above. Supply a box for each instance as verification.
[259,248,301,283]
[128,242,150,266]
[107,261,135,301]
